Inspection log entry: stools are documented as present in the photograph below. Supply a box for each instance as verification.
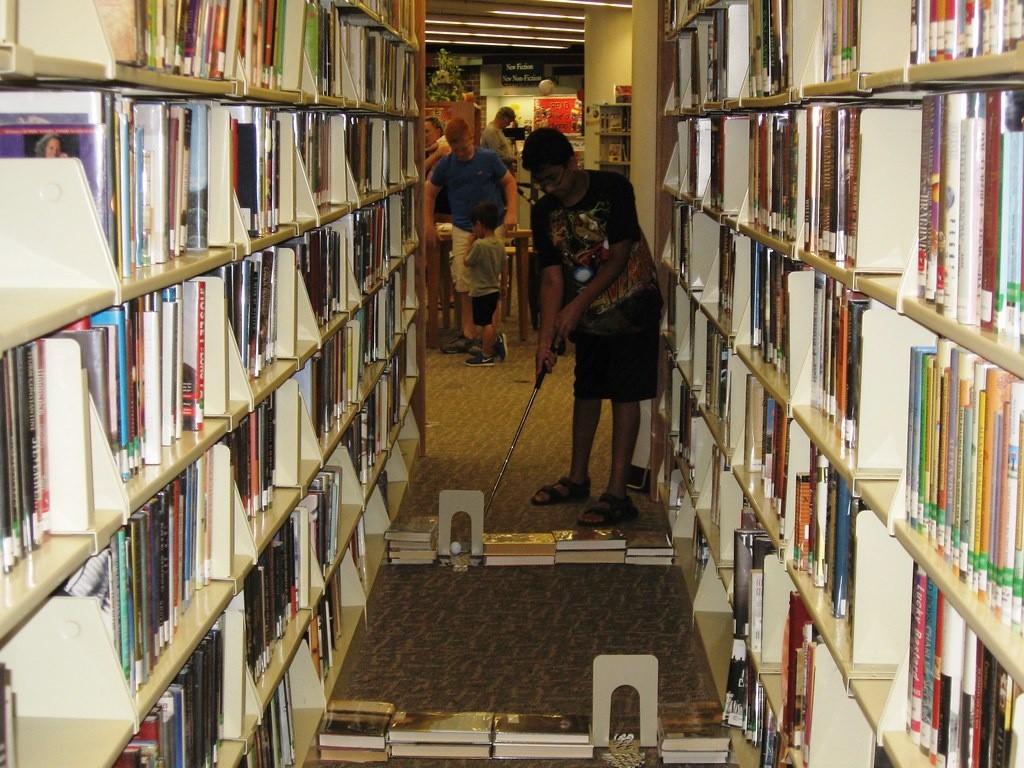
[500,246,536,321]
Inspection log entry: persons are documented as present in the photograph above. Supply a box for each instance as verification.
[425,134,451,159]
[521,127,664,528]
[424,118,519,354]
[480,106,515,179]
[425,116,456,311]
[462,202,509,366]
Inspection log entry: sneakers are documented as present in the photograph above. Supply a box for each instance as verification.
[492,334,508,361]
[465,353,494,366]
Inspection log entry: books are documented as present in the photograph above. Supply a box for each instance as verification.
[0,0,1024,768]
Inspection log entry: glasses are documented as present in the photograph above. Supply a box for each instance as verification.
[530,161,569,189]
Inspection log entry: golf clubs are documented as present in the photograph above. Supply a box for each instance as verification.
[484,326,560,517]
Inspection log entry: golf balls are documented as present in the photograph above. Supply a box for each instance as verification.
[450,540,462,556]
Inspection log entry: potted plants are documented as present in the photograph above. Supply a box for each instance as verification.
[428,48,463,101]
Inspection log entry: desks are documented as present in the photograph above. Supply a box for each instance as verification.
[427,229,532,349]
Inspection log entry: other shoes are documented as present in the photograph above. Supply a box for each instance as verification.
[467,338,482,353]
[443,336,470,353]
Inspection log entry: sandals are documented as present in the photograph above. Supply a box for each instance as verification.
[530,476,591,505]
[576,492,640,528]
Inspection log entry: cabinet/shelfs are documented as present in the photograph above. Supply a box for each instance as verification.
[656,0,1023,768]
[425,100,482,146]
[0,0,427,768]
[594,103,631,184]
